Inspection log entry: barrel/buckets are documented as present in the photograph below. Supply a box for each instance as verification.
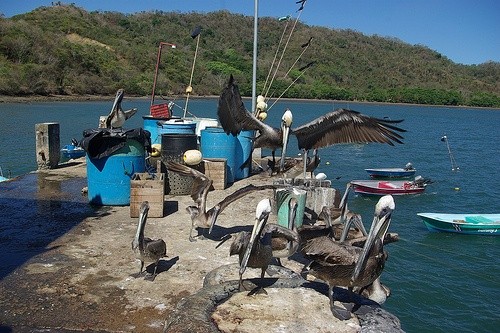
[142,117,165,145]
[162,124,196,136]
[161,133,197,196]
[201,129,236,183]
[113,139,144,154]
[86,154,147,206]
[275,187,307,230]
[205,127,254,180]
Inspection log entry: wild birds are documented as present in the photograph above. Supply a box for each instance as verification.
[105,88,139,132]
[230,198,301,299]
[287,182,400,320]
[132,201,169,282]
[166,160,277,242]
[217,74,407,175]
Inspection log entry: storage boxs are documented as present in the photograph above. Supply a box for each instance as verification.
[130,171,166,218]
[190,158,228,189]
[151,102,170,119]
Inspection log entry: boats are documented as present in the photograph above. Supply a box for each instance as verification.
[365,161,416,179]
[417,213,500,237]
[350,175,430,197]
[59,144,86,160]
